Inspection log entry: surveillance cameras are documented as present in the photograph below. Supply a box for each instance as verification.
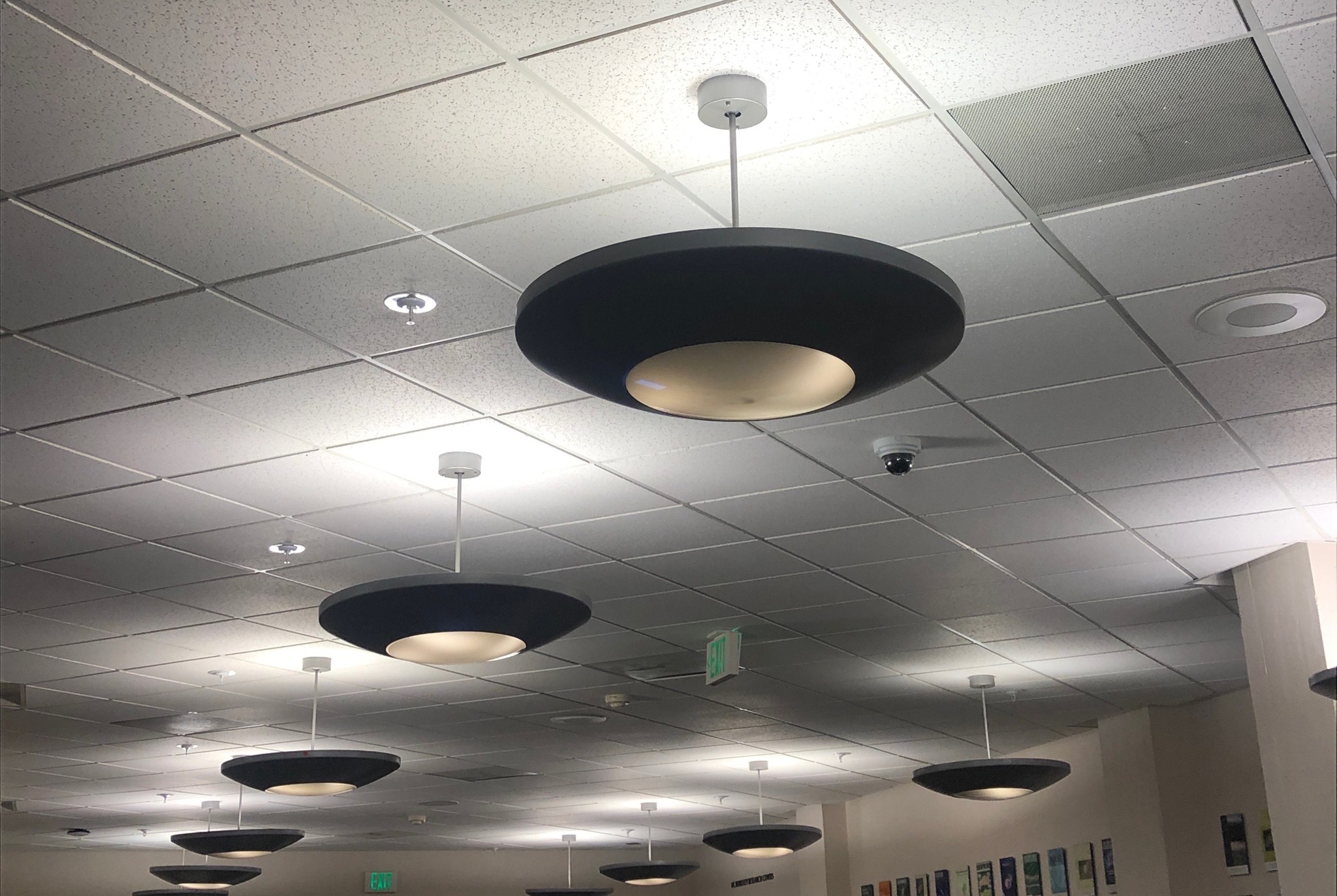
[872,435,922,476]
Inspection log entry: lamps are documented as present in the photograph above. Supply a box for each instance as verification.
[131,800,262,896]
[702,759,823,861]
[514,73,966,422]
[317,451,593,665]
[221,656,402,797]
[912,675,1074,802]
[171,755,306,859]
[525,802,702,896]
